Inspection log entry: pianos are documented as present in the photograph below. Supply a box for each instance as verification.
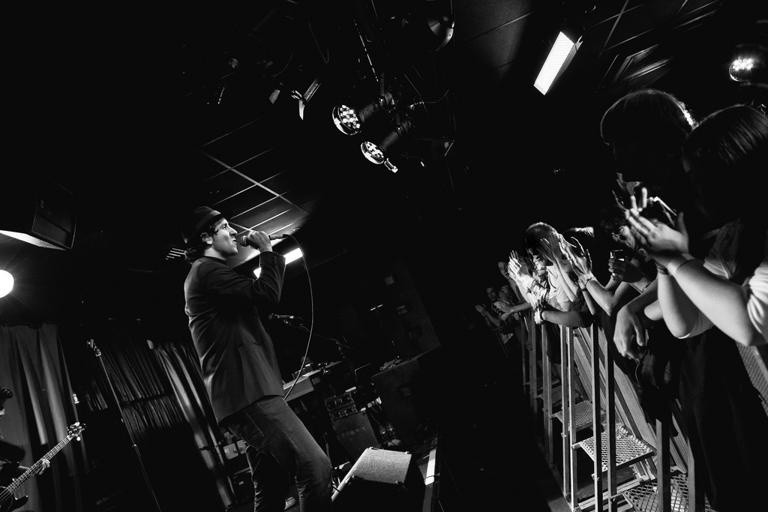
[283,358,344,400]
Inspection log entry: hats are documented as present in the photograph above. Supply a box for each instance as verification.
[180,205,221,245]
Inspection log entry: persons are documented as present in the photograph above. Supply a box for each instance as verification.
[180,203,336,511]
[0,386,52,512]
[474,86,767,512]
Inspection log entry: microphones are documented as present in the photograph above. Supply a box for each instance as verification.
[239,232,286,247]
[268,312,295,321]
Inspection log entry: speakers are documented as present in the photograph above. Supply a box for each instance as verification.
[226,454,254,507]
[371,344,442,442]
[330,447,425,512]
[0,176,80,251]
[288,362,344,455]
[330,411,381,458]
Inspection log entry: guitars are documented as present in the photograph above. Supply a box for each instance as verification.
[1,422,87,511]
